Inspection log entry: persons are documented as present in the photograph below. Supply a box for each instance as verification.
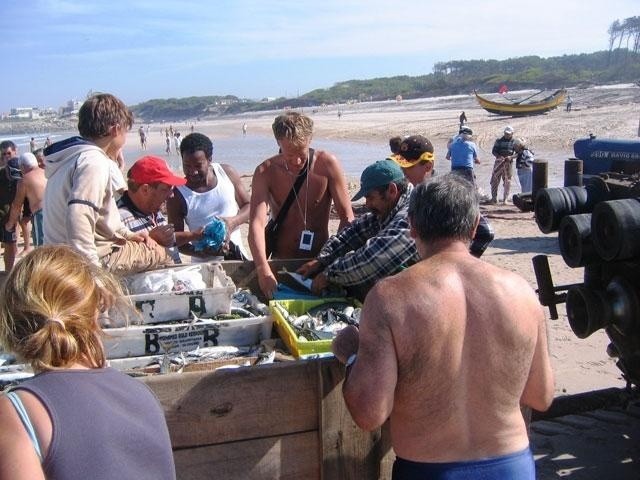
[5,152,48,249]
[113,155,187,247]
[44,92,167,313]
[460,112,467,128]
[0,157,23,276]
[113,149,124,172]
[388,134,494,258]
[0,244,176,480]
[390,138,402,152]
[166,134,170,153]
[33,148,46,168]
[243,123,248,135]
[248,113,354,302]
[513,138,534,194]
[446,131,480,165]
[174,134,180,156]
[160,120,197,134]
[166,134,252,262]
[0,140,30,257]
[30,136,36,152]
[44,137,53,148]
[448,129,478,190]
[296,159,423,305]
[567,95,572,111]
[138,125,145,150]
[486,127,514,204]
[177,133,182,141]
[332,173,556,480]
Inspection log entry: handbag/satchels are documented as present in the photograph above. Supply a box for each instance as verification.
[265,148,314,259]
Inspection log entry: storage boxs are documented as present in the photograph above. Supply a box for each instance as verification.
[102,262,236,327]
[127,354,397,480]
[100,292,274,362]
[268,298,363,360]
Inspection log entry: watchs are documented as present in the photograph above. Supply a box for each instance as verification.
[344,352,356,371]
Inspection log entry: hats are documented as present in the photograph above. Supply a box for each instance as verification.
[31,146,44,153]
[127,156,187,186]
[463,128,473,135]
[8,157,22,179]
[460,126,469,130]
[504,126,513,134]
[351,161,404,201]
[386,135,434,168]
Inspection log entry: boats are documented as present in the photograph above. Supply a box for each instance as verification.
[469,78,572,115]
[475,82,568,115]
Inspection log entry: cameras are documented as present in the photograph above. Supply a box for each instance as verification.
[299,230,314,251]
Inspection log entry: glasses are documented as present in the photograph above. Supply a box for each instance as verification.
[2,151,12,155]
